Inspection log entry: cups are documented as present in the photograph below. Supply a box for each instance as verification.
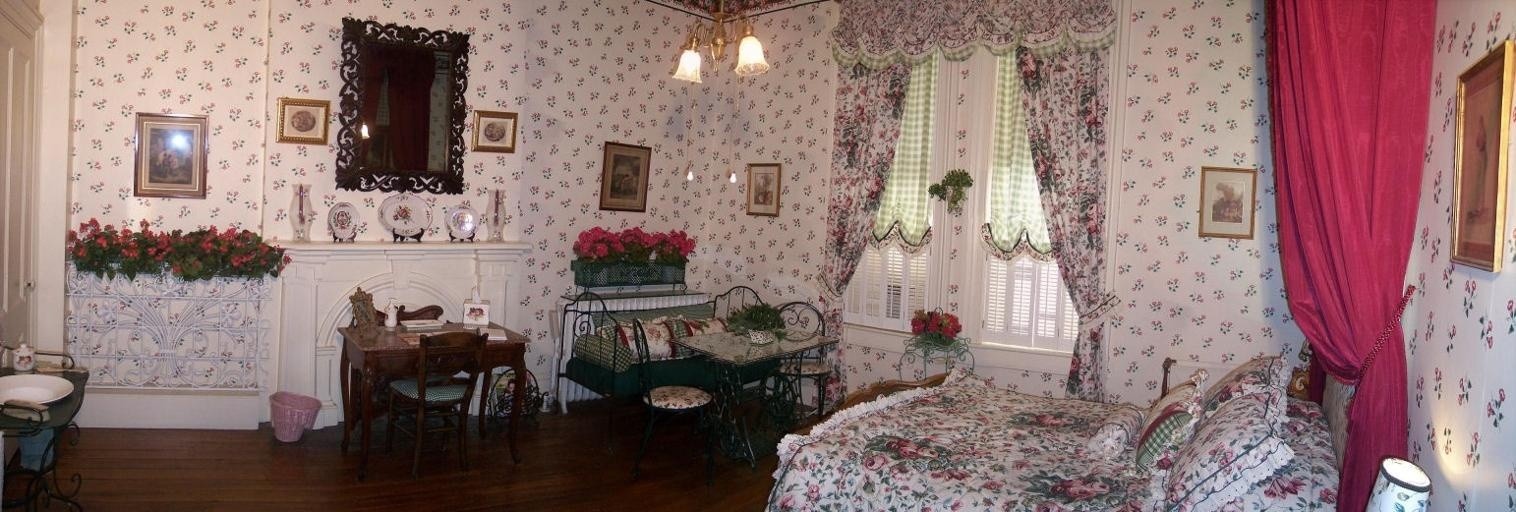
[13,342,35,371]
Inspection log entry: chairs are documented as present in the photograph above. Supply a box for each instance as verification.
[331,285,532,480]
[623,317,713,486]
[778,302,826,422]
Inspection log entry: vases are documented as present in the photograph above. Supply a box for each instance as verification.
[485,189,507,241]
[289,183,314,240]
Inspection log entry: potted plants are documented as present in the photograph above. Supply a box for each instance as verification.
[727,302,783,345]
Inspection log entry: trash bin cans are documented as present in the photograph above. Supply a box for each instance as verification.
[269,392,322,443]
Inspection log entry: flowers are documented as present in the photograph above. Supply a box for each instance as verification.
[910,307,964,347]
[570,224,696,286]
[64,218,291,283]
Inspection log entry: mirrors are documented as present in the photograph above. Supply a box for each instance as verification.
[332,14,471,195]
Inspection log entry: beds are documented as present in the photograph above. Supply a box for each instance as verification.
[763,367,1358,511]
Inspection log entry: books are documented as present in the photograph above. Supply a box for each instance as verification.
[398,330,462,346]
[477,326,508,341]
[399,319,442,332]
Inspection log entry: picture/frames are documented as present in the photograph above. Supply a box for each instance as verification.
[1197,166,1260,241]
[1448,39,1514,273]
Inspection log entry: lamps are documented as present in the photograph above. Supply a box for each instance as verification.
[1363,455,1436,512]
[670,2,771,84]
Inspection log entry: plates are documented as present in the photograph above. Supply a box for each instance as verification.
[327,202,360,241]
[444,205,481,240]
[377,193,432,238]
[0,372,74,405]
[400,319,443,327]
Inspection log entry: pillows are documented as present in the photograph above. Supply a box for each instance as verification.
[1090,352,1292,511]
[572,333,632,373]
[670,313,728,359]
[597,314,696,364]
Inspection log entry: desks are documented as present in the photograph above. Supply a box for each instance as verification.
[665,324,839,469]
[1,346,89,512]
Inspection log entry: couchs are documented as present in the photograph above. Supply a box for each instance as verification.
[555,286,765,451]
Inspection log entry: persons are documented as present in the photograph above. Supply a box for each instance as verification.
[503,378,517,414]
